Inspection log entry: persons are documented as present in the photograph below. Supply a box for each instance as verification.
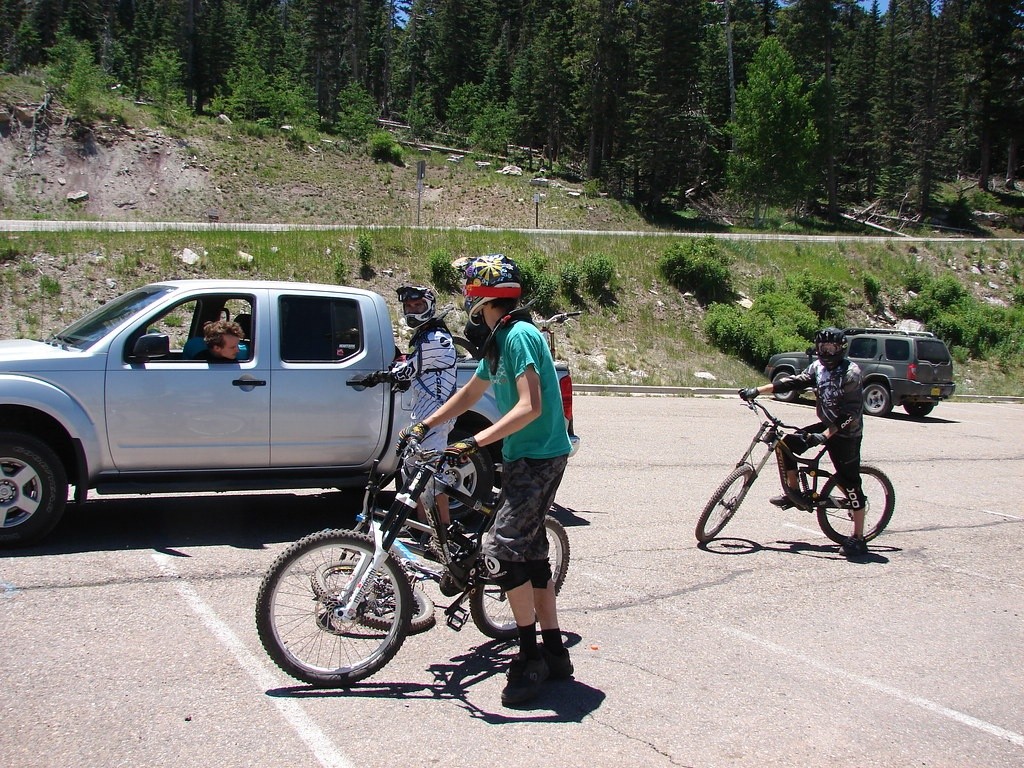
[740,328,868,555]
[395,254,574,704]
[192,320,243,359]
[362,286,450,527]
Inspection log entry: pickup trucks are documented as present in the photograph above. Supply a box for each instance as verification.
[1,280,582,523]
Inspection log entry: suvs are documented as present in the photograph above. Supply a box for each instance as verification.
[765,328,957,417]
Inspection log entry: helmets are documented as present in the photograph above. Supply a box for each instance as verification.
[461,254,521,326]
[396,284,436,328]
[814,327,848,369]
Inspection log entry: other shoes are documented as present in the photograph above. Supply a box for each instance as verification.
[419,532,430,550]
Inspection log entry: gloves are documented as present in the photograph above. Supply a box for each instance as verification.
[804,433,827,448]
[436,435,481,468]
[396,421,430,457]
[391,380,411,393]
[363,371,380,387]
[738,388,760,401]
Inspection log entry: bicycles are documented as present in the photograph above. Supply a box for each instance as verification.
[310,458,482,635]
[250,432,595,693]
[692,388,896,548]
[446,309,581,365]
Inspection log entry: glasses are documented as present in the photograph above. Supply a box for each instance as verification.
[818,342,840,355]
[398,291,424,301]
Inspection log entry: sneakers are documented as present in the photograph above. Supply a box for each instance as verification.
[770,489,802,506]
[846,535,867,562]
[501,655,550,705]
[506,648,574,678]
